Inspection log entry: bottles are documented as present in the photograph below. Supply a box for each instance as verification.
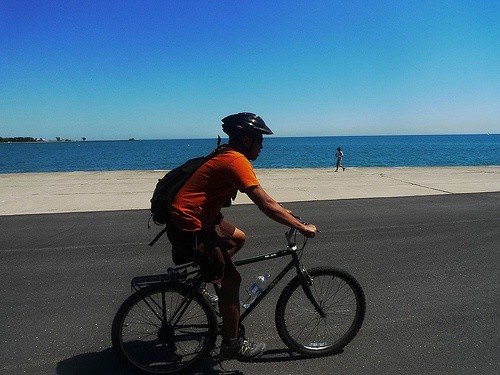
[240,272,270,308]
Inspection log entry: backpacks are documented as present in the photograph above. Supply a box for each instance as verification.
[149,146,232,227]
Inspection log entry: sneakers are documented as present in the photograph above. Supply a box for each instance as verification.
[220,338,266,360]
[196,285,219,305]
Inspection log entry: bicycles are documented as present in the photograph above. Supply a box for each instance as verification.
[111,211,367,375]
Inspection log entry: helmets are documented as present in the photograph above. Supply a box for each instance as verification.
[221,112,273,136]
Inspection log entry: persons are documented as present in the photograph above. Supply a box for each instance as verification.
[334,147,346,172]
[165,113,319,359]
